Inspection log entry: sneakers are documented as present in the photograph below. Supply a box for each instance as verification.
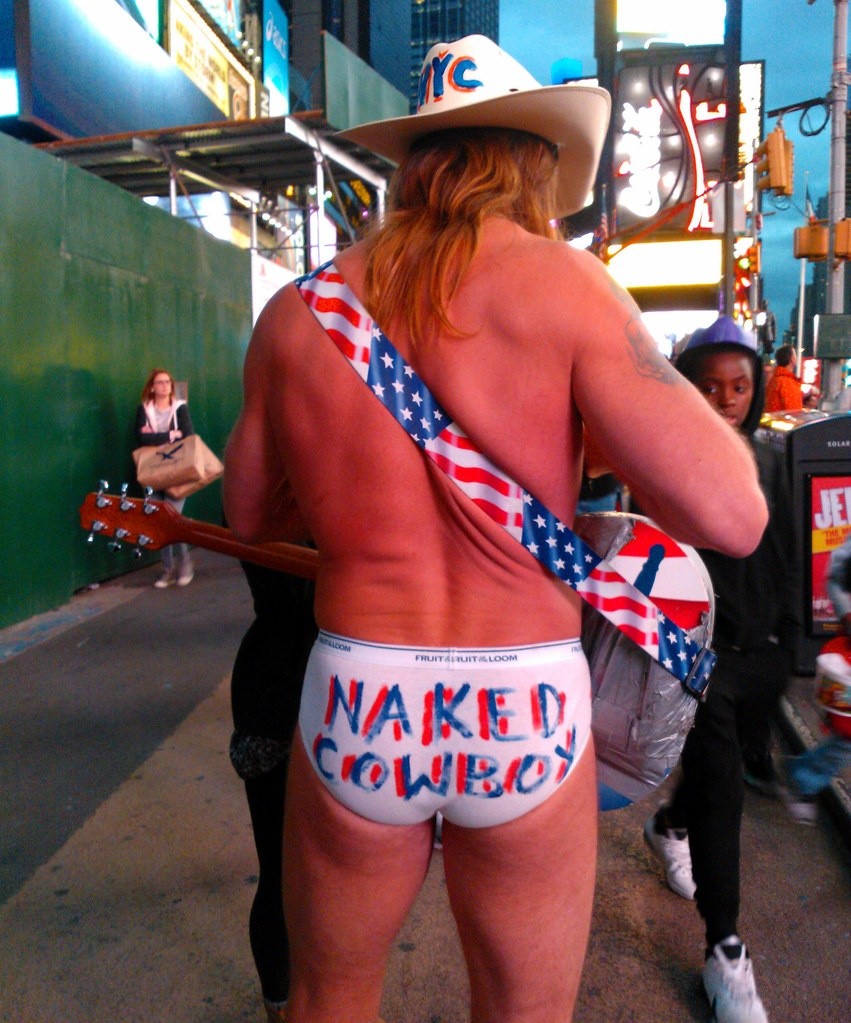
[700,941,771,1023]
[643,803,697,902]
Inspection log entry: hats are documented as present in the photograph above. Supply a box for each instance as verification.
[334,33,613,219]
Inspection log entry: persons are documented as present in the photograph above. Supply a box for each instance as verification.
[221,31,769,1022]
[643,313,818,1023]
[779,533,850,829]
[228,546,341,1023]
[127,368,196,589]
[765,345,803,411]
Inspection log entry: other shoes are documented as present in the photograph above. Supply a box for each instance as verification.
[177,571,194,586]
[154,574,177,589]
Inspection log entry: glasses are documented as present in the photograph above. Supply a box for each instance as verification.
[152,380,170,384]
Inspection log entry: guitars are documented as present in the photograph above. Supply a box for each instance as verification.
[78,479,717,813]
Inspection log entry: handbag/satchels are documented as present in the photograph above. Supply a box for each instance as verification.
[131,435,204,490]
[164,440,225,499]
